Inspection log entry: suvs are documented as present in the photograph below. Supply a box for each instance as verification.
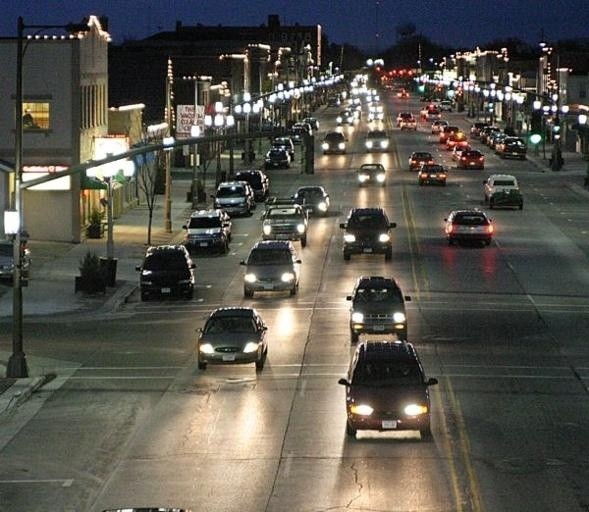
[339,208,396,263]
[347,276,412,343]
[259,196,308,247]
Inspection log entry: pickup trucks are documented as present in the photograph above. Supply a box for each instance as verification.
[483,173,524,209]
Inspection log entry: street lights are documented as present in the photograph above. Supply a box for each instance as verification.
[543,94,571,170]
[188,124,205,207]
[421,76,544,160]
[267,73,346,141]
[162,76,174,232]
[4,195,32,379]
[236,92,261,163]
[160,134,181,233]
[7,16,91,377]
[577,109,589,152]
[454,75,567,171]
[190,77,201,207]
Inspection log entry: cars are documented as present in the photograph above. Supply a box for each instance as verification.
[471,122,528,161]
[395,99,451,131]
[431,119,485,169]
[265,118,346,168]
[356,164,386,187]
[419,164,447,187]
[196,308,267,372]
[327,75,383,125]
[444,208,494,249]
[409,151,432,171]
[0,242,32,285]
[291,185,330,216]
[363,129,390,153]
[236,170,270,200]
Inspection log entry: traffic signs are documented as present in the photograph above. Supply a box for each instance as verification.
[235,69,346,168]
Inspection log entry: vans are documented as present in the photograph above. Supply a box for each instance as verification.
[240,241,301,299]
[135,245,197,301]
[210,181,255,217]
[338,341,439,440]
[183,209,233,257]
